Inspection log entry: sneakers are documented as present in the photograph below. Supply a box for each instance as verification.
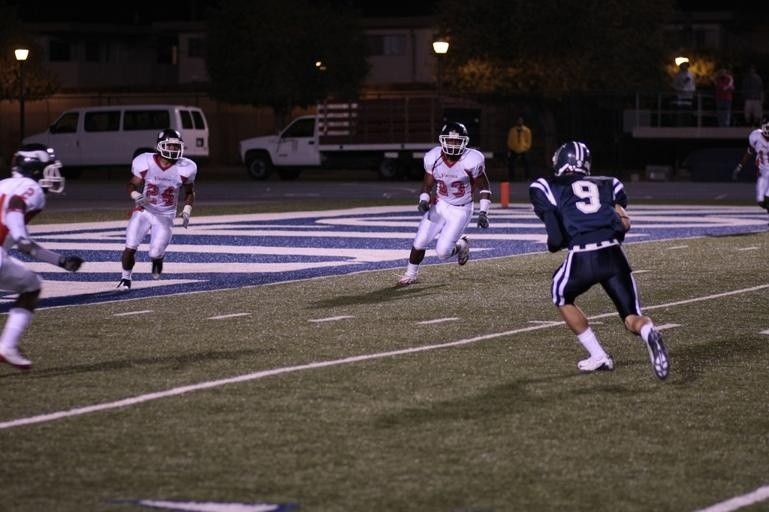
[399,273,417,286]
[151,257,163,279]
[1,343,33,366]
[645,327,669,380]
[457,237,469,265]
[115,279,131,291]
[578,354,614,371]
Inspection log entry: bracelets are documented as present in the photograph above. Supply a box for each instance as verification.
[419,192,431,204]
[182,204,192,217]
[737,164,743,171]
[130,191,141,200]
[479,198,491,213]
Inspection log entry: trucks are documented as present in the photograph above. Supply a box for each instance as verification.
[239,91,495,181]
[21,104,212,181]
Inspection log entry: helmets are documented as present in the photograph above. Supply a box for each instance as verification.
[438,121,470,160]
[552,141,592,180]
[11,144,65,194]
[157,129,184,161]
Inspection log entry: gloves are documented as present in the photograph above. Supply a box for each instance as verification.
[59,255,84,271]
[135,194,148,208]
[418,200,429,212]
[478,211,489,228]
[179,212,189,227]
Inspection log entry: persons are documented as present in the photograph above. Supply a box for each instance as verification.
[0,144,84,369]
[528,138,671,381]
[714,64,735,127]
[671,61,697,123]
[731,113,768,213]
[505,115,533,182]
[397,120,493,286]
[116,129,199,294]
[742,64,764,124]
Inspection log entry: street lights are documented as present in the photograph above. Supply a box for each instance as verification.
[14,41,31,140]
[674,43,690,94]
[431,20,450,89]
